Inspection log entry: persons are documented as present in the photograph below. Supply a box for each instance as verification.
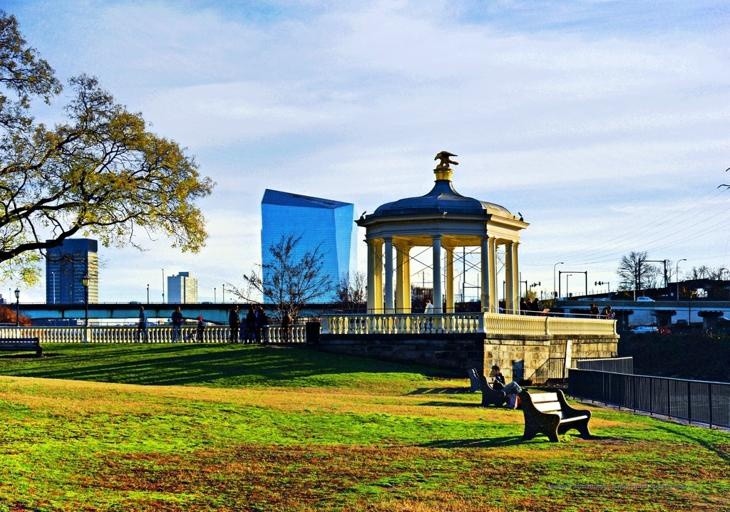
[228,303,271,345]
[282,311,293,342]
[423,300,433,314]
[589,302,616,319]
[196,316,206,343]
[488,365,505,390]
[519,296,540,316]
[172,306,185,343]
[134,306,148,341]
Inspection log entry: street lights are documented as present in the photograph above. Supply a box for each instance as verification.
[554,262,564,299]
[14,287,21,326]
[676,259,687,301]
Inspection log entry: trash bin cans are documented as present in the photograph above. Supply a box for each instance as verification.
[305,322,321,346]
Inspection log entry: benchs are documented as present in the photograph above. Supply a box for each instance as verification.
[0,337,43,357]
[468,368,592,443]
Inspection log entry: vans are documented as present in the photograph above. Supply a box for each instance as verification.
[637,297,655,302]
[630,326,659,334]
